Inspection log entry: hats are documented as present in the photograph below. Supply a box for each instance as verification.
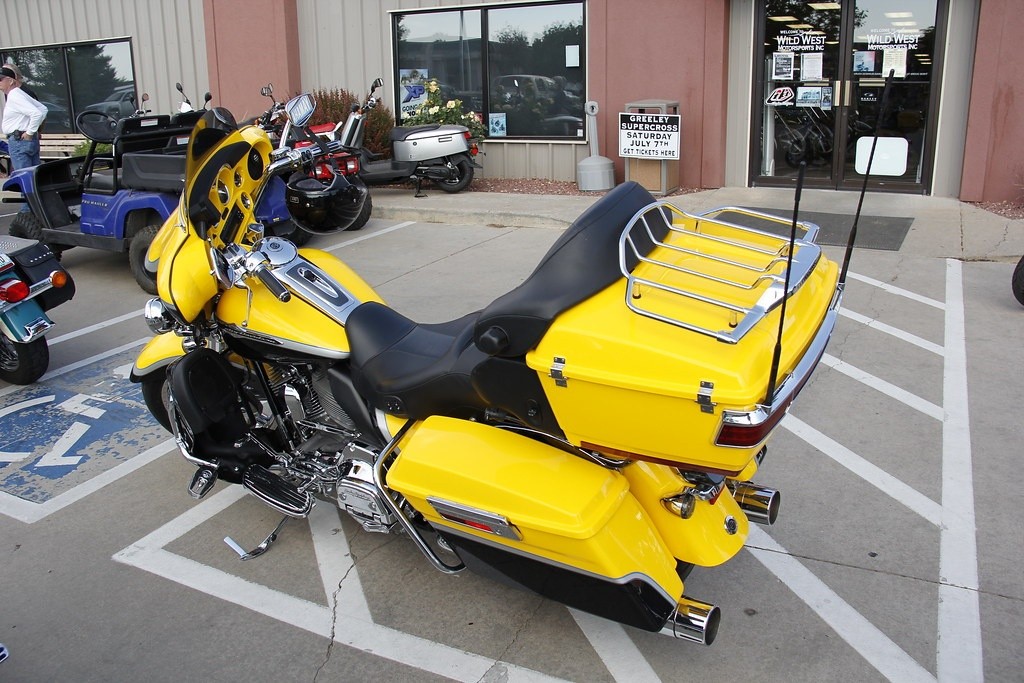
[0,67,15,80]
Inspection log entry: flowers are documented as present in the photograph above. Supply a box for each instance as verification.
[401,77,488,157]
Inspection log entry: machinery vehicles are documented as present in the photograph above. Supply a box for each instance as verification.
[0,101,315,297]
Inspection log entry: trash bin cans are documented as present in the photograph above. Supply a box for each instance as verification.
[625,99,681,197]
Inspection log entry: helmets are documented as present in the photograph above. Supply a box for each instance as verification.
[285,173,368,235]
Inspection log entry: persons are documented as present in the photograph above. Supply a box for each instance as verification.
[0,63,50,211]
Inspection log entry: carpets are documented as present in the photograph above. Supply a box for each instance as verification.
[713,207,916,251]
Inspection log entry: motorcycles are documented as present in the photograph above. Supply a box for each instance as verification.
[128,94,846,647]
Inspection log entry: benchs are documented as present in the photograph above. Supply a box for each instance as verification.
[0,133,92,177]
[83,114,176,189]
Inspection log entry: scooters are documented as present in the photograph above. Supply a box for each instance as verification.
[0,237,76,388]
[250,80,482,232]
[769,96,875,173]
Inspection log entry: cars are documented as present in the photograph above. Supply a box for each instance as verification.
[492,75,579,104]
[42,102,71,130]
